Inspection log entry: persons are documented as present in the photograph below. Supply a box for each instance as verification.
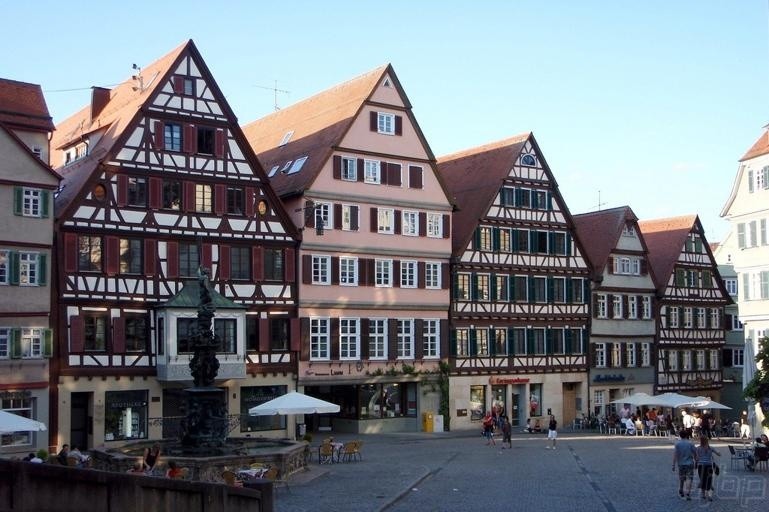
[127,461,148,476]
[58,443,70,465]
[739,409,754,441]
[533,418,543,434]
[544,415,559,452]
[23,452,36,462]
[745,434,768,471]
[525,417,534,434]
[591,402,731,441]
[164,460,189,479]
[79,450,90,465]
[745,436,763,448]
[671,428,695,498]
[498,417,513,449]
[144,443,161,470]
[196,263,216,313]
[692,435,723,502]
[482,412,499,446]
[30,455,43,463]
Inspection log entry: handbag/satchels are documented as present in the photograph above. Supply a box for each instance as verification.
[713,462,719,475]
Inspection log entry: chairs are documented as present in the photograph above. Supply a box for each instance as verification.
[306,440,363,464]
[223,463,293,492]
[728,441,769,473]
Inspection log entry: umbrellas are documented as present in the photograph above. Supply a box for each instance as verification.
[610,393,656,420]
[1,411,47,435]
[249,387,331,432]
[641,392,693,419]
[684,396,730,417]
